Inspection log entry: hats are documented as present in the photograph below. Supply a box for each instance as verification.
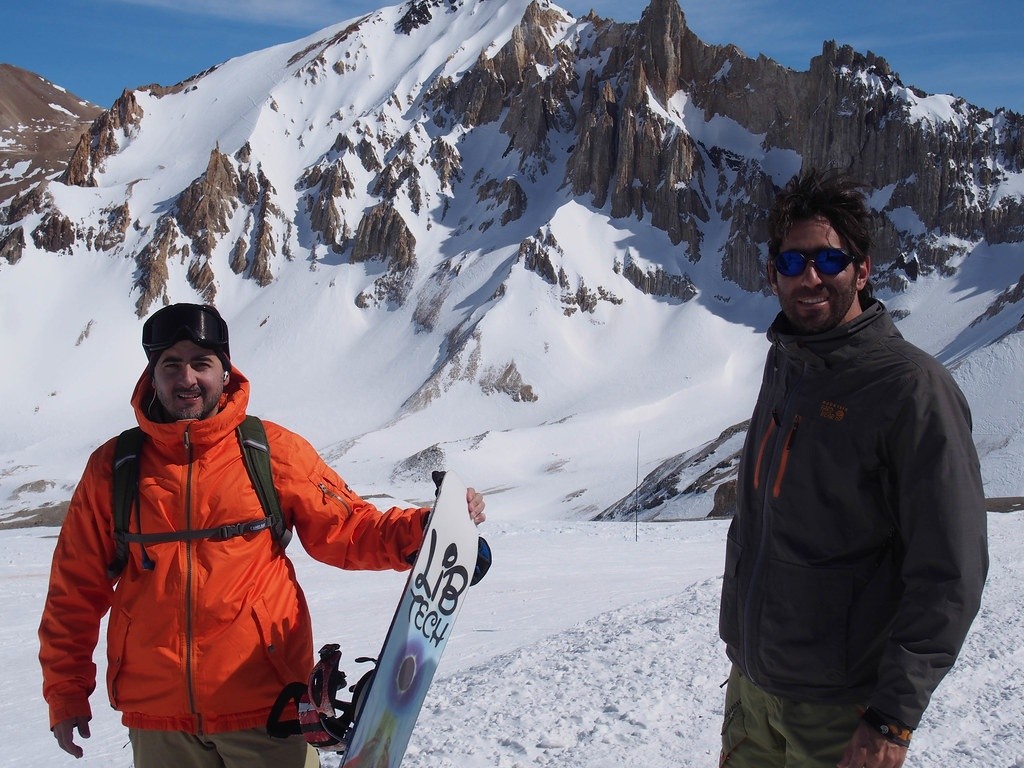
[140,300,233,376]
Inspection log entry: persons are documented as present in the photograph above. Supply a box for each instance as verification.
[717,168,991,768]
[38,302,487,768]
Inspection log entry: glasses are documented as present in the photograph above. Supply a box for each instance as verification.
[142,305,229,358]
[772,246,864,277]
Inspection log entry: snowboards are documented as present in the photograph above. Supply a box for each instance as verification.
[337,469,480,768]
